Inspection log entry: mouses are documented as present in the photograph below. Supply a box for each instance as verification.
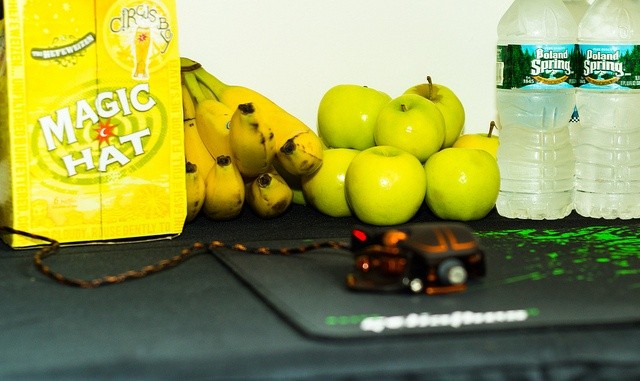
[342,221,486,294]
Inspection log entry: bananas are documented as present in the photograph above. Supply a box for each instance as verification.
[180,54,323,217]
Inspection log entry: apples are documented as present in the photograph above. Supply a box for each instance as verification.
[300,148,361,217]
[344,145,427,227]
[452,121,501,160]
[404,76,466,146]
[424,146,501,222]
[317,83,393,149]
[373,93,446,162]
[288,185,305,205]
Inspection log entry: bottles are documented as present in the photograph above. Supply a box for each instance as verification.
[497,1,577,219]
[576,0,640,220]
